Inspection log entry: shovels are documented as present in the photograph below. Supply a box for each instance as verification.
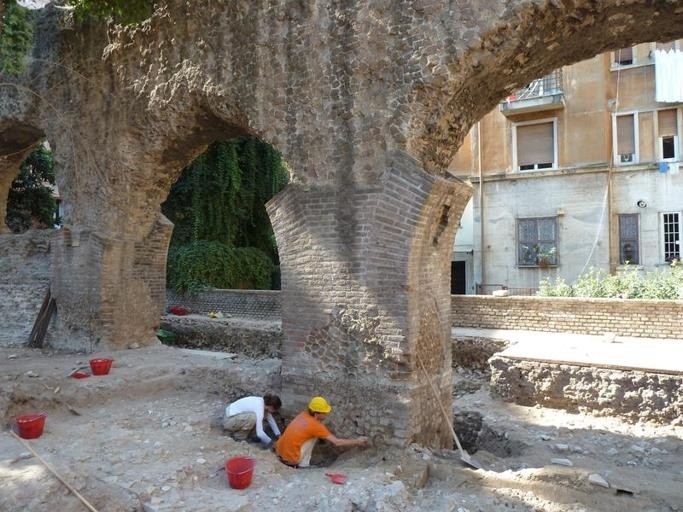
[417,354,483,469]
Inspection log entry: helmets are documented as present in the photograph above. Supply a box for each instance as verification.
[308,396,331,414]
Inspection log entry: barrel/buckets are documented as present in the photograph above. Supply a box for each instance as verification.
[225,457,254,488]
[15,413,46,438]
[89,358,112,375]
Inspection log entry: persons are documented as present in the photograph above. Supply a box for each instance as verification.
[222,396,368,467]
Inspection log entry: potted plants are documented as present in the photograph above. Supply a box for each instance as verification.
[521,244,557,267]
